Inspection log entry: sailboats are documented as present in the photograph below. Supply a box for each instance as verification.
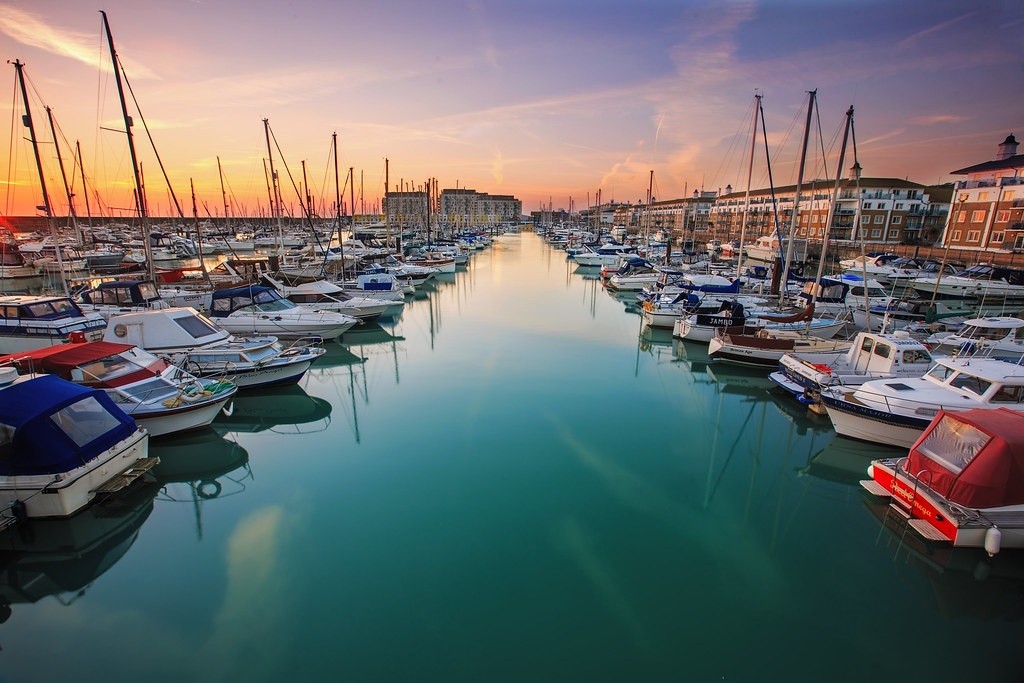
[0,9,505,538]
[535,85,1024,557]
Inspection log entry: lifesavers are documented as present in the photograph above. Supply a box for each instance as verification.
[177,379,204,402]
[365,281,376,291]
